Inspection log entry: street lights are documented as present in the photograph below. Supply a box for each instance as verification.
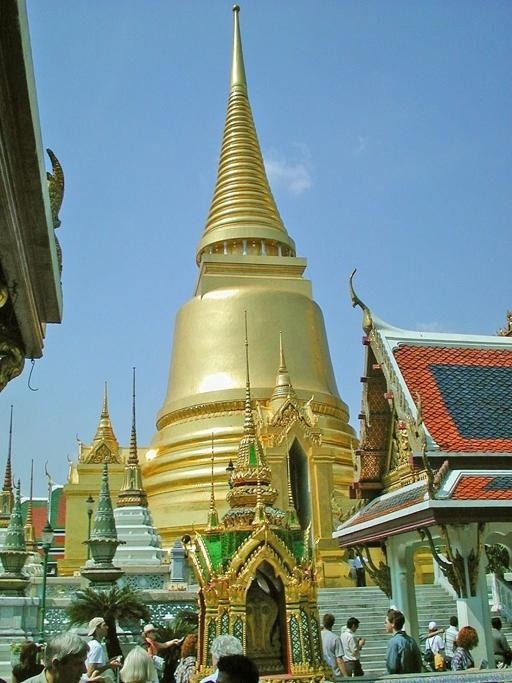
[224,456,236,489]
[84,492,96,561]
[38,518,55,645]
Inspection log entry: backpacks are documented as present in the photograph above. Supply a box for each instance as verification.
[424,648,434,662]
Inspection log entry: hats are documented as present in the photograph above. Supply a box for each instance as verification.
[88,617,105,637]
[429,621,437,630]
[142,624,157,637]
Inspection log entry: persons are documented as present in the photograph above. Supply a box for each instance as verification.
[341,617,365,676]
[443,616,459,669]
[384,609,420,674]
[354,554,369,587]
[80,617,122,682]
[120,624,198,682]
[320,614,349,677]
[425,622,444,672]
[11,641,45,683]
[451,627,481,671]
[23,632,90,683]
[200,634,259,682]
[492,618,510,666]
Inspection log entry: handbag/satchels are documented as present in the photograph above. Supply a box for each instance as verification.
[443,656,452,668]
